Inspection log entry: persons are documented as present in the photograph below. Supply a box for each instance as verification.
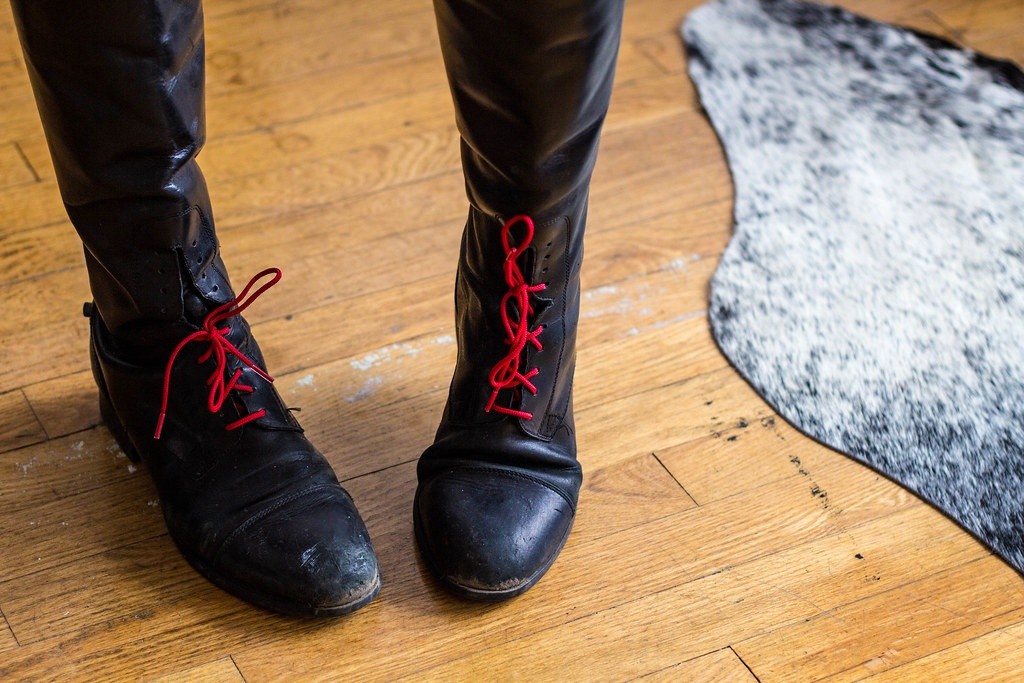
[10,0,625,622]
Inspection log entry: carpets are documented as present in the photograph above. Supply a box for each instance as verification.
[682,0,1024,580]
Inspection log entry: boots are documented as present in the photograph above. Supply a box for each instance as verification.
[9,0,379,620]
[412,0,627,607]
